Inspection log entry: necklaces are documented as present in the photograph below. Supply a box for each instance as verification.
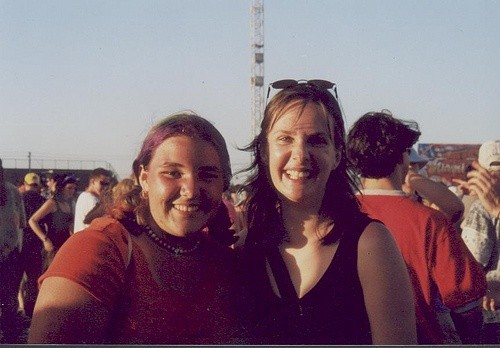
[138,208,202,253]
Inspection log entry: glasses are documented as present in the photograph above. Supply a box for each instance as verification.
[95,181,110,186]
[265,78,338,109]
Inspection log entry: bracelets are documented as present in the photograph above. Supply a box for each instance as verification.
[41,235,48,243]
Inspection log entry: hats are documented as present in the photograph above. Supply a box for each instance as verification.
[25,172,40,185]
[478,140,500,172]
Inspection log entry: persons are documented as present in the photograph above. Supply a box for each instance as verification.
[73,168,114,234]
[84,178,136,224]
[460,141,500,344]
[468,160,500,239]
[401,148,465,225]
[44,173,59,199]
[20,174,53,317]
[0,159,27,330]
[28,113,263,345]
[235,83,418,345]
[28,175,78,266]
